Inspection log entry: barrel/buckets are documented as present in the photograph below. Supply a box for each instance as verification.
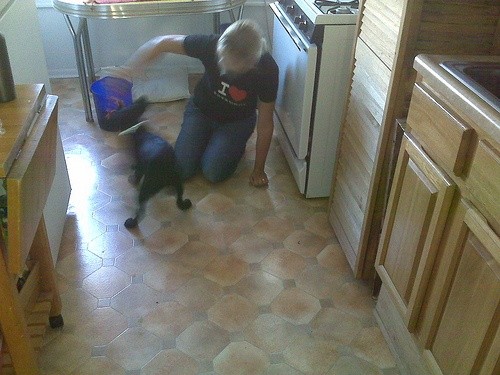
[90,66,134,133]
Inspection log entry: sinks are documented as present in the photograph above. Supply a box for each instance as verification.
[447,60,500,112]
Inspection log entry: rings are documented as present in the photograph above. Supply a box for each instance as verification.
[262,178,265,181]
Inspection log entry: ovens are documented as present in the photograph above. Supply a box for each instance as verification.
[269,1,355,198]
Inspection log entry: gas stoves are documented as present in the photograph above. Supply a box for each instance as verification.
[276,0,360,44]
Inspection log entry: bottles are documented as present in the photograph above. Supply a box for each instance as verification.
[0,34,16,103]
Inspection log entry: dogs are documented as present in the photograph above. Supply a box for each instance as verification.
[99,94,194,230]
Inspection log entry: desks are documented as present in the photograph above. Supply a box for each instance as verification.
[0,83,64,375]
[52,0,242,123]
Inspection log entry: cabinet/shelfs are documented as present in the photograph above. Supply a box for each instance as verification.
[373,74,500,375]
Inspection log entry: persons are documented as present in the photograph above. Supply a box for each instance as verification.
[125,18,279,187]
